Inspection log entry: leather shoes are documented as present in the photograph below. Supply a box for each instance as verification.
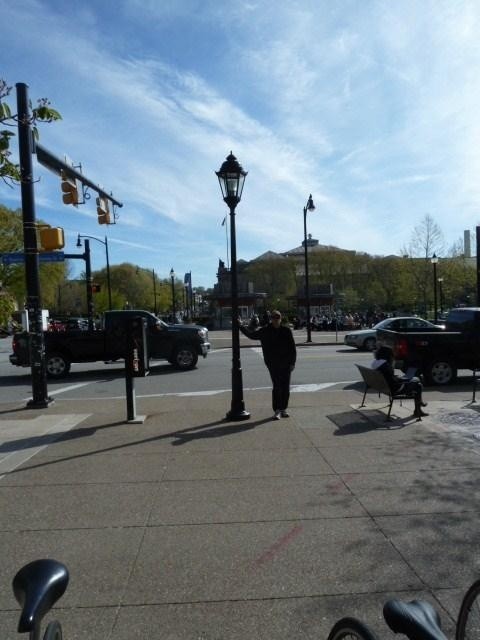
[414,401,429,416]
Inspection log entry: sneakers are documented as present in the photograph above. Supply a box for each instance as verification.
[273,409,289,420]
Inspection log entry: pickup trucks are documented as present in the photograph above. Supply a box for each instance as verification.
[374,305,480,386]
[9,309,210,378]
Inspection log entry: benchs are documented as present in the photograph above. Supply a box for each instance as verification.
[353,362,423,423]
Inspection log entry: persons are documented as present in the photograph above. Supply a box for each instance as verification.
[239,308,397,331]
[238,308,297,420]
[371,344,428,418]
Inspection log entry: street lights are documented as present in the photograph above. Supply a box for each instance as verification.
[76,233,114,310]
[302,193,315,344]
[431,253,444,324]
[170,267,176,323]
[213,150,252,422]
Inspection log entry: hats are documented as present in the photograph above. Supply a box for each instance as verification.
[271,311,281,317]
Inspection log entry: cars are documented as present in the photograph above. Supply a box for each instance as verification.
[342,316,447,352]
